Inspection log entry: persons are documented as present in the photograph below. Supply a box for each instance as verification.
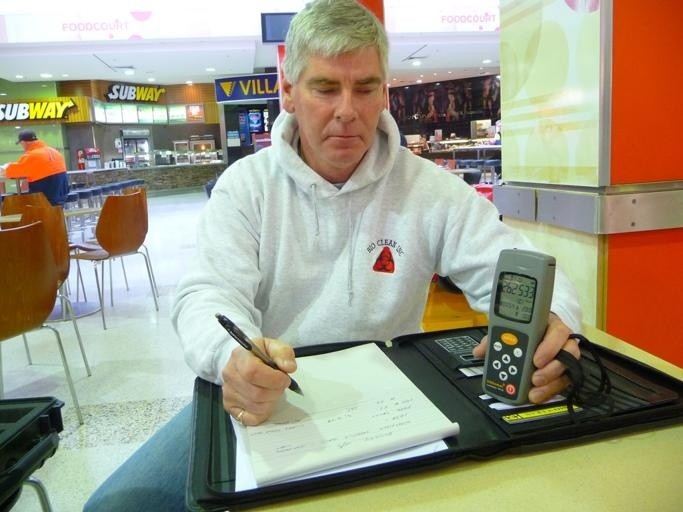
[0,129,70,207]
[387,74,501,123]
[81,2,587,511]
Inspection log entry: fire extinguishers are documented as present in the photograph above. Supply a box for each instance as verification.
[77,149,84,169]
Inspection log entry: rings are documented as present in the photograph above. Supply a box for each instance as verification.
[234,409,243,420]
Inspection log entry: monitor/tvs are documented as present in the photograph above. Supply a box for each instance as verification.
[261,12,298,43]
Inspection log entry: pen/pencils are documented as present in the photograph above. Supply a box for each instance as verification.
[215,313,305,396]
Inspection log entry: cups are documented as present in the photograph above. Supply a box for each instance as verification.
[104,160,127,169]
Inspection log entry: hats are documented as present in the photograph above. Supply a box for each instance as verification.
[16,129,37,144]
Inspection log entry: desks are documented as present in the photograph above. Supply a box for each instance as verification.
[227,322,683,511]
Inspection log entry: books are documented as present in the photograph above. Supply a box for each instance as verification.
[234,343,462,487]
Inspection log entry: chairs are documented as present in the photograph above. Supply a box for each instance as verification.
[434,158,457,170]
[0,184,160,427]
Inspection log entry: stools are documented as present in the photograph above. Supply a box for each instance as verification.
[65,179,146,232]
[444,159,501,185]
